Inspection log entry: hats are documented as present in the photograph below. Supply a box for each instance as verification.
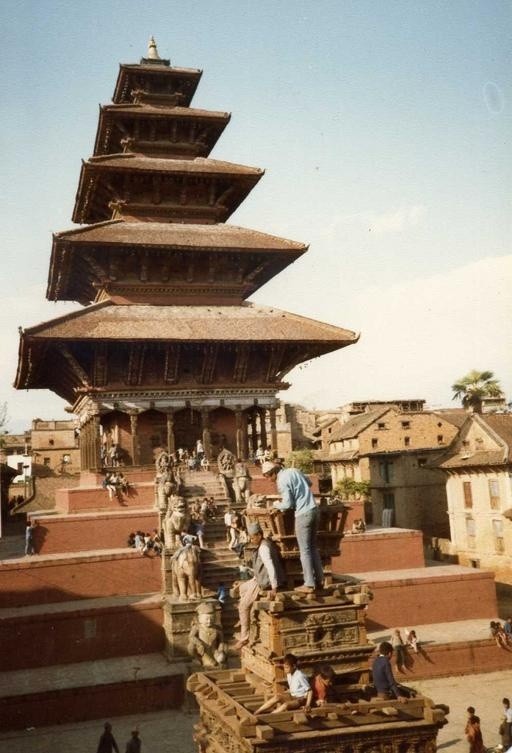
[261,462,278,475]
[247,521,261,534]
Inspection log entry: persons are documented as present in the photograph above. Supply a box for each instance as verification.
[252,651,315,717]
[496,622,508,645]
[125,724,142,751]
[100,444,129,500]
[227,520,289,650]
[256,445,274,464]
[8,494,26,509]
[310,665,337,706]
[23,520,39,556]
[191,497,238,551]
[466,715,485,752]
[261,461,325,594]
[352,519,365,534]
[502,616,512,643]
[464,704,477,734]
[216,582,227,603]
[499,696,511,752]
[407,628,419,653]
[95,720,121,752]
[127,529,162,555]
[489,619,503,648]
[370,638,409,706]
[177,440,210,472]
[391,628,407,673]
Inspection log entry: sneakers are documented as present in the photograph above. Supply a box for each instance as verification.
[294,584,314,593]
